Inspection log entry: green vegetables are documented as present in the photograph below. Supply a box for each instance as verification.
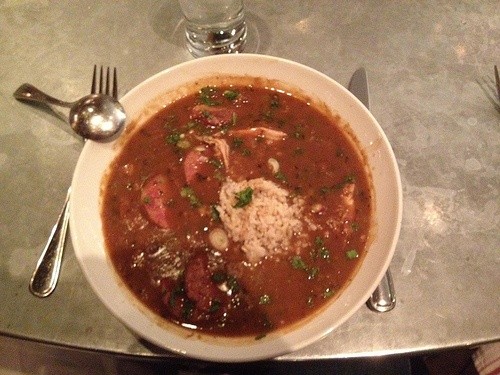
[143,87,361,341]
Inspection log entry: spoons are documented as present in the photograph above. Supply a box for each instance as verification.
[12,82,125,139]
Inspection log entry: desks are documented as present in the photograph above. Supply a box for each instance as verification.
[0,0,500,375]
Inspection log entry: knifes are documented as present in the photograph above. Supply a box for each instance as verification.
[346,68,396,313]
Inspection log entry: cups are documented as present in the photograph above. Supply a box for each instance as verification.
[172,0,259,61]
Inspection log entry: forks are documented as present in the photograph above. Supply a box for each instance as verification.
[30,64,119,298]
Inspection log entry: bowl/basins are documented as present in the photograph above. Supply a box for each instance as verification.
[68,53,401,364]
